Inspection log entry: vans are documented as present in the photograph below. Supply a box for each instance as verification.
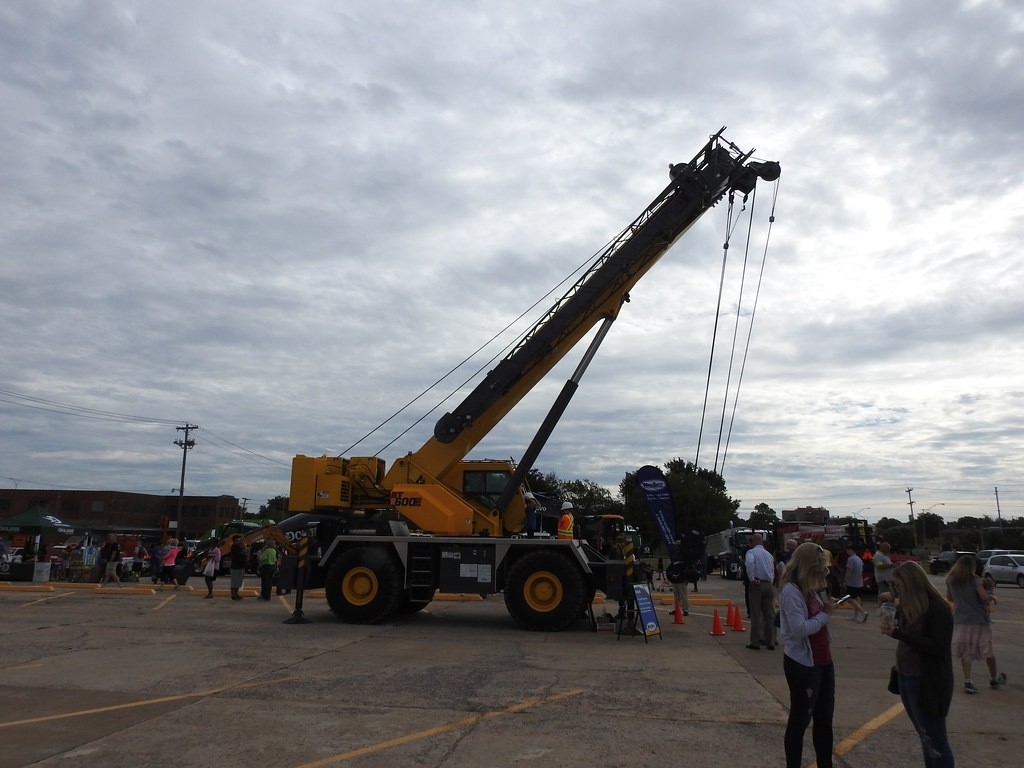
[3,547,23,562]
[51,545,98,567]
[976,549,1024,572]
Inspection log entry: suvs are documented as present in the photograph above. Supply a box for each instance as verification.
[930,551,976,574]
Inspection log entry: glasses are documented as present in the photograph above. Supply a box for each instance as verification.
[894,583,904,591]
[818,546,823,553]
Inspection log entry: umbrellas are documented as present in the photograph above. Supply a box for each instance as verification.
[0,505,75,562]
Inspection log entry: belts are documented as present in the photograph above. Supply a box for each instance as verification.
[755,578,770,582]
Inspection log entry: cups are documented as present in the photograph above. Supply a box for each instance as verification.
[880,602,896,635]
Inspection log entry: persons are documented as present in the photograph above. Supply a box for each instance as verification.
[100,533,191,589]
[693,532,832,650]
[256,540,277,600]
[0,537,9,561]
[229,535,248,601]
[872,542,894,613]
[204,540,221,599]
[597,522,619,560]
[778,543,842,768]
[557,503,574,541]
[880,561,955,768]
[655,556,666,581]
[522,492,538,538]
[945,556,1007,693]
[842,545,869,623]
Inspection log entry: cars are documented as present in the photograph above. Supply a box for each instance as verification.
[982,554,1024,589]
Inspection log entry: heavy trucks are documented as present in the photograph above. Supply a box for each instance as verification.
[703,520,774,579]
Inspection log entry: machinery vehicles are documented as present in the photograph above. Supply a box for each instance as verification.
[844,518,921,596]
[577,513,651,600]
[173,524,325,591]
[285,124,781,632]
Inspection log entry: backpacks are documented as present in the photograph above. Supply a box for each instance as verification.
[137,546,146,559]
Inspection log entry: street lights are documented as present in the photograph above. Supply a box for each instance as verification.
[922,504,945,560]
[854,507,871,519]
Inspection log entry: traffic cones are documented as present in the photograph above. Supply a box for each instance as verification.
[709,608,725,635]
[731,607,746,631]
[724,601,736,626]
[672,601,684,624]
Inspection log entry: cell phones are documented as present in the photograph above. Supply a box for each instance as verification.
[835,594,851,606]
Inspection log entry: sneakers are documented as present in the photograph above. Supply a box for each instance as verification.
[990,672,1007,689]
[964,682,979,693]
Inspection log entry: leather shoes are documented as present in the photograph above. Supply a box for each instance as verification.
[767,645,775,650]
[746,644,761,650]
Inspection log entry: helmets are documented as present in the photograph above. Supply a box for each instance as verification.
[560,502,574,510]
[523,492,535,499]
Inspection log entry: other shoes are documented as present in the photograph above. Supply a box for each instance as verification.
[862,611,869,622]
[846,615,860,621]
[669,610,675,615]
[257,596,271,600]
[663,578,666,581]
[155,588,164,592]
[230,594,242,602]
[174,585,180,591]
[683,611,689,616]
[204,595,214,599]
[656,578,661,580]
